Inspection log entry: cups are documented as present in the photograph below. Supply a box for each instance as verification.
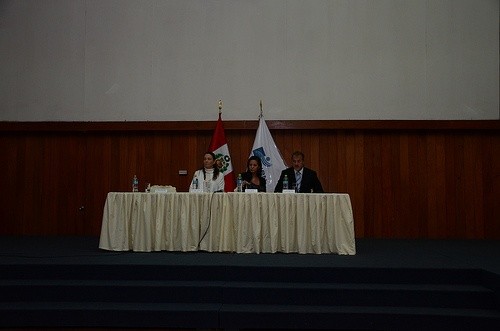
[145,183,151,193]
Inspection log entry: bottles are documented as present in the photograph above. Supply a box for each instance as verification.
[283,175,289,190]
[192,175,198,189]
[237,174,243,192]
[132,175,138,192]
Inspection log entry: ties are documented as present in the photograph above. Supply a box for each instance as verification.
[296,172,301,192]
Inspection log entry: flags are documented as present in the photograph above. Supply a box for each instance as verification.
[247,116,289,193]
[209,116,236,192]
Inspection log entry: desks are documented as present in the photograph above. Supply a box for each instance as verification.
[99,190,356,252]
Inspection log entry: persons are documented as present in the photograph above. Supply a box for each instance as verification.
[237,156,266,193]
[274,151,324,193]
[189,151,224,192]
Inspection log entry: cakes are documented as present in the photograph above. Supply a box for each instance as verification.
[150,185,177,192]
